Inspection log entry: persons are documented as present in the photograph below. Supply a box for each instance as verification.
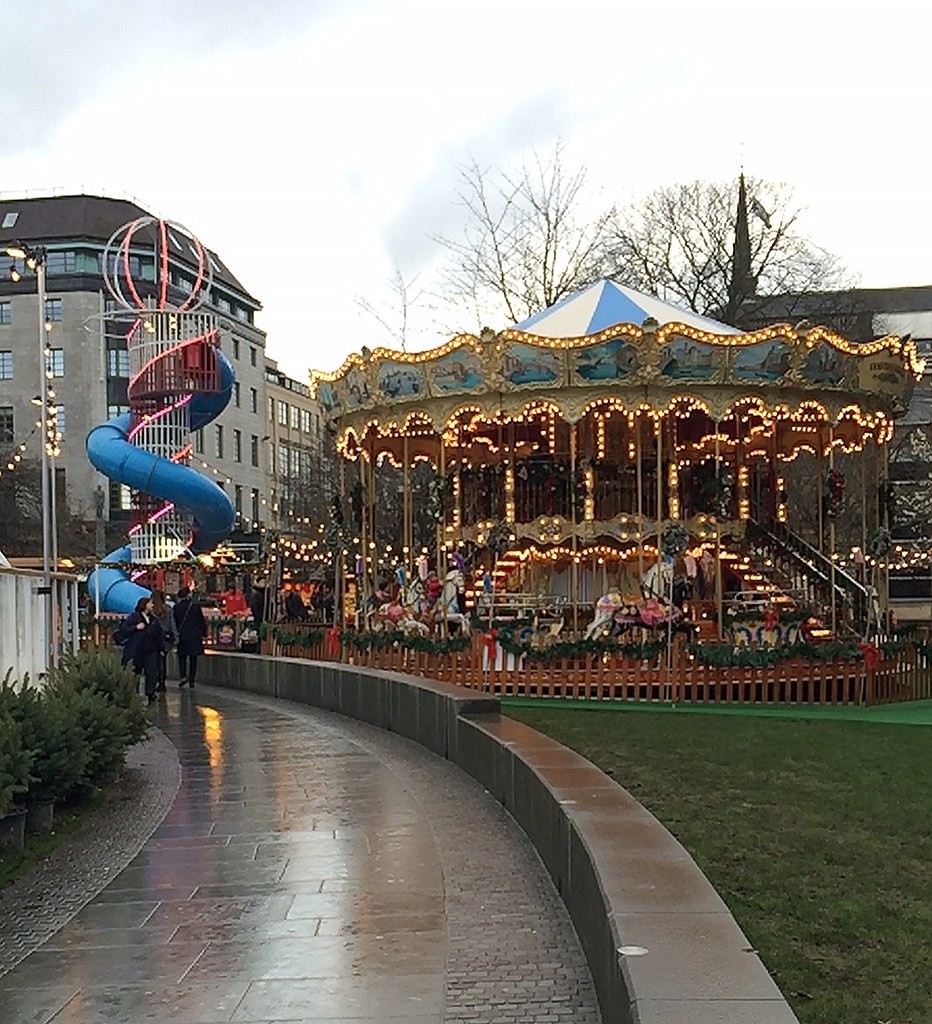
[150,591,178,691]
[280,588,309,622]
[214,582,247,615]
[249,577,274,625]
[173,586,207,687]
[121,597,163,702]
[882,607,895,627]
[94,486,104,517]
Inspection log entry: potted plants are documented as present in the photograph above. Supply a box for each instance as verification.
[1,643,155,853]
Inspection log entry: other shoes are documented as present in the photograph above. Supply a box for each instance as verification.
[156,685,166,689]
[178,677,186,687]
[189,683,193,687]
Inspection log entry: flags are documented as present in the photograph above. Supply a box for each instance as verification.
[754,200,772,229]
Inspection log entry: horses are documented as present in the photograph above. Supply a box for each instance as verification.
[370,555,696,646]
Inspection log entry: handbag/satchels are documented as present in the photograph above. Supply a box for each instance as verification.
[173,630,181,645]
[113,610,147,646]
[162,628,176,643]
